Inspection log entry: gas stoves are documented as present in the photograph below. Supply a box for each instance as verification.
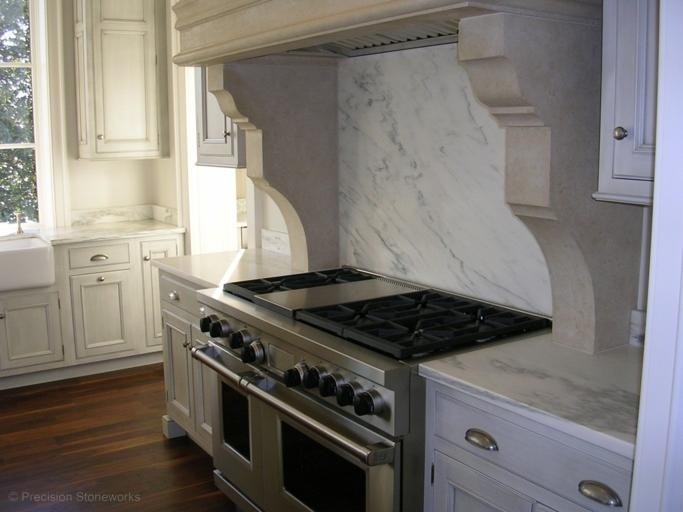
[223,266,552,360]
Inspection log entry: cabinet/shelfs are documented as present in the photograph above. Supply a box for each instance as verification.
[0,285,77,390]
[158,269,213,459]
[424,379,627,511]
[52,235,184,379]
[192,67,244,167]
[592,1,658,207]
[75,0,166,157]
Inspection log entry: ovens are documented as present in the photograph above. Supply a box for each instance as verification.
[191,302,412,512]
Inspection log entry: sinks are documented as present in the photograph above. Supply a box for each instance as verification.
[0,233,55,290]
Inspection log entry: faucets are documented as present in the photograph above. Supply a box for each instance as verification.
[15,212,23,234]
